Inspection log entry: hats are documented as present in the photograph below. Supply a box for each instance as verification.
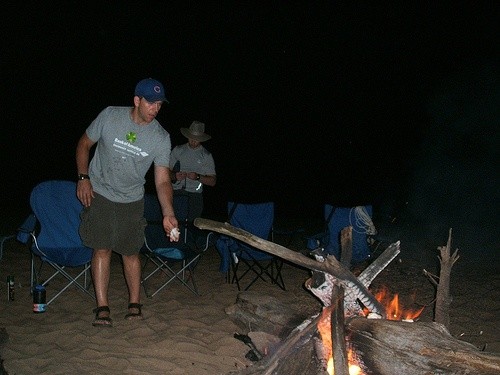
[180,122,210,141]
[135,77,170,105]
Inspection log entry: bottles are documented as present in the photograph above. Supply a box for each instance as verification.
[33,285,46,313]
[7,276,14,302]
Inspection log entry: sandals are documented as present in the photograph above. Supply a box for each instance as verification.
[124,302,144,321]
[92,306,113,328]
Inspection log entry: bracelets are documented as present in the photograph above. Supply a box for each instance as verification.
[77,174,91,180]
[195,174,201,181]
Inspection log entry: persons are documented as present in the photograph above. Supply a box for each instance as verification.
[169,121,216,220]
[76,77,178,328]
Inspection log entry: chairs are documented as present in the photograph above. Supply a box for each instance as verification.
[215,200,286,291]
[142,194,212,299]
[18,180,96,305]
[305,204,373,267]
